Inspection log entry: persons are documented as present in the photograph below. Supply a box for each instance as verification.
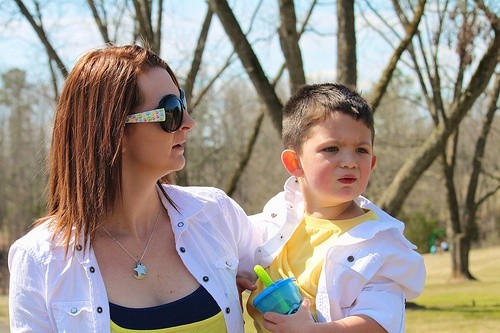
[6,45,307,333]
[236,83,426,333]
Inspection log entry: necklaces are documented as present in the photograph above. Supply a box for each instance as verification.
[95,203,163,280]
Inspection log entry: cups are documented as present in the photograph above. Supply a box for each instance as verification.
[254,276,316,329]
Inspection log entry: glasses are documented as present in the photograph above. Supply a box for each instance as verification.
[125,87,188,134]
